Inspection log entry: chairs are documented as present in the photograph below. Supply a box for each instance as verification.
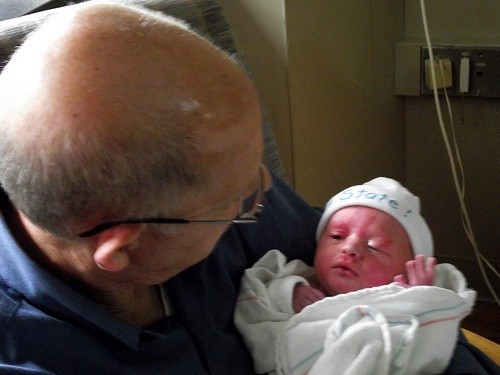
[0,0,500,375]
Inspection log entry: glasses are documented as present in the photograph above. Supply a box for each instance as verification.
[77,166,265,238]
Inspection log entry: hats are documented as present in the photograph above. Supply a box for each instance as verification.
[316,177,434,266]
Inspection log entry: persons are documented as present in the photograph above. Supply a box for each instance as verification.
[0,0,500,375]
[232,176,478,375]
